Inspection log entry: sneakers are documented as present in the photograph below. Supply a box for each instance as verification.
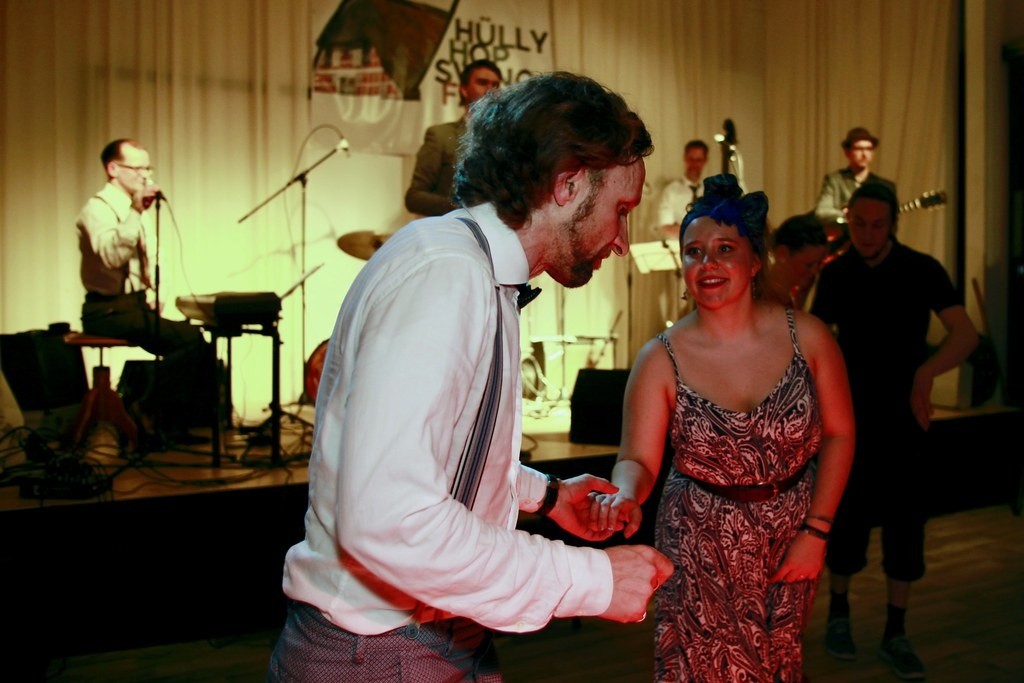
[879,636,928,678]
[825,617,856,658]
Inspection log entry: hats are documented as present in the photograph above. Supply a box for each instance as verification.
[839,127,880,151]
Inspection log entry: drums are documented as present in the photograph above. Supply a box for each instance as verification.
[306,339,334,403]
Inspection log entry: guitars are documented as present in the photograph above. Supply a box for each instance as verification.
[822,187,947,265]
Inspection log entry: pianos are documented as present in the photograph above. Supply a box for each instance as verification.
[175,290,281,469]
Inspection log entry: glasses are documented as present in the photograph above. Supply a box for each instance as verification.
[120,166,152,173]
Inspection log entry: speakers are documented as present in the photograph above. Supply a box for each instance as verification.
[116,358,228,427]
[568,368,632,446]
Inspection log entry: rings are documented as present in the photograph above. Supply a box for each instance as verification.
[800,574,805,580]
[636,612,646,623]
[654,578,660,591]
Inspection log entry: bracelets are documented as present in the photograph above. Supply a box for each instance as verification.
[534,474,559,516]
[801,524,829,541]
[805,514,833,523]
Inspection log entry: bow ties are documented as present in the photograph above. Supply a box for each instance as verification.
[515,282,542,310]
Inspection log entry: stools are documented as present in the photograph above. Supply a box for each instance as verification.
[65,328,142,463]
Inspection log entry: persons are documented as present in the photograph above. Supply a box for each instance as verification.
[577,173,855,683]
[656,140,709,322]
[812,128,880,227]
[809,169,980,677]
[405,58,503,216]
[76,139,212,453]
[754,211,837,334]
[272,71,674,683]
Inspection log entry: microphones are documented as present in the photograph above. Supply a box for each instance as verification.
[143,179,166,201]
[341,137,351,157]
[715,135,738,152]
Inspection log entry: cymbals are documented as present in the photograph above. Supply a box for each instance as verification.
[338,232,395,261]
